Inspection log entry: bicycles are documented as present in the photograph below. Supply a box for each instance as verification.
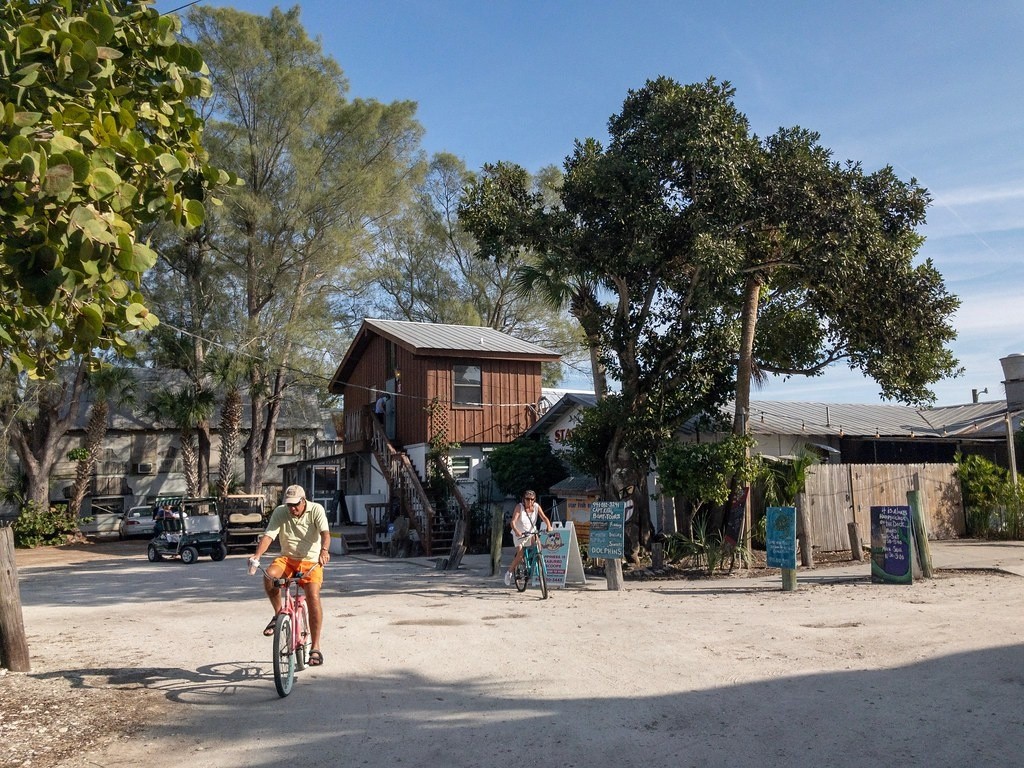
[247,560,314,697]
[513,528,553,599]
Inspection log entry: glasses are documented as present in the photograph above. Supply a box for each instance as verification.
[524,498,535,501]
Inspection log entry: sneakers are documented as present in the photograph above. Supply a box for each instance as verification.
[504,570,512,585]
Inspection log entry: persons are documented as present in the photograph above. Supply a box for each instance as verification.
[504,488,554,586]
[374,393,391,440]
[248,483,331,667]
[154,505,188,520]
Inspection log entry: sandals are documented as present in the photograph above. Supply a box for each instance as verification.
[263,616,278,636]
[309,649,323,666]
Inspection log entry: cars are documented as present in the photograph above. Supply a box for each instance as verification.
[117,506,157,540]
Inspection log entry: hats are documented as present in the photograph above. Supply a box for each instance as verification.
[282,485,306,505]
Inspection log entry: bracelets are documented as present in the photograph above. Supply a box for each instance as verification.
[321,547,328,550]
